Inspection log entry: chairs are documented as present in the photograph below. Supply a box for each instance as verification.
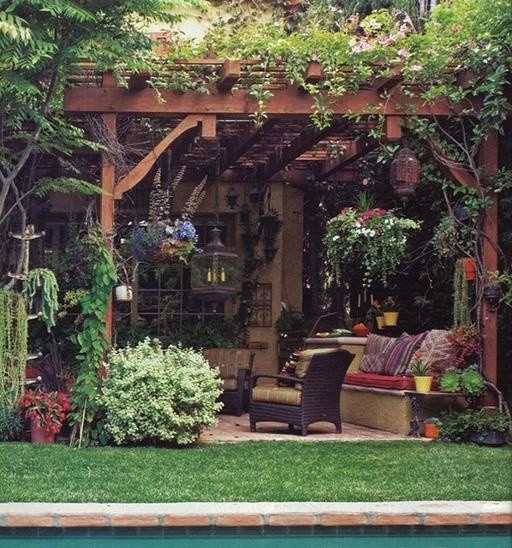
[198,348,355,436]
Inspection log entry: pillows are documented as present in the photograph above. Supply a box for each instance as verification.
[359,328,464,379]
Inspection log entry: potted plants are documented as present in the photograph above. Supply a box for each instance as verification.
[430,216,476,280]
[399,353,509,447]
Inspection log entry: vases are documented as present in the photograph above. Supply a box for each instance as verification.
[374,311,399,330]
[26,419,59,446]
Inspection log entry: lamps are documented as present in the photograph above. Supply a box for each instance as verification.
[190,141,239,313]
[389,129,422,209]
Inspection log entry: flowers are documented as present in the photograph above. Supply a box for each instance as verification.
[16,385,72,441]
[318,188,424,289]
[371,296,402,316]
[126,163,209,269]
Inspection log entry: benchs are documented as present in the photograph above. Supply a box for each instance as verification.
[337,345,458,436]
